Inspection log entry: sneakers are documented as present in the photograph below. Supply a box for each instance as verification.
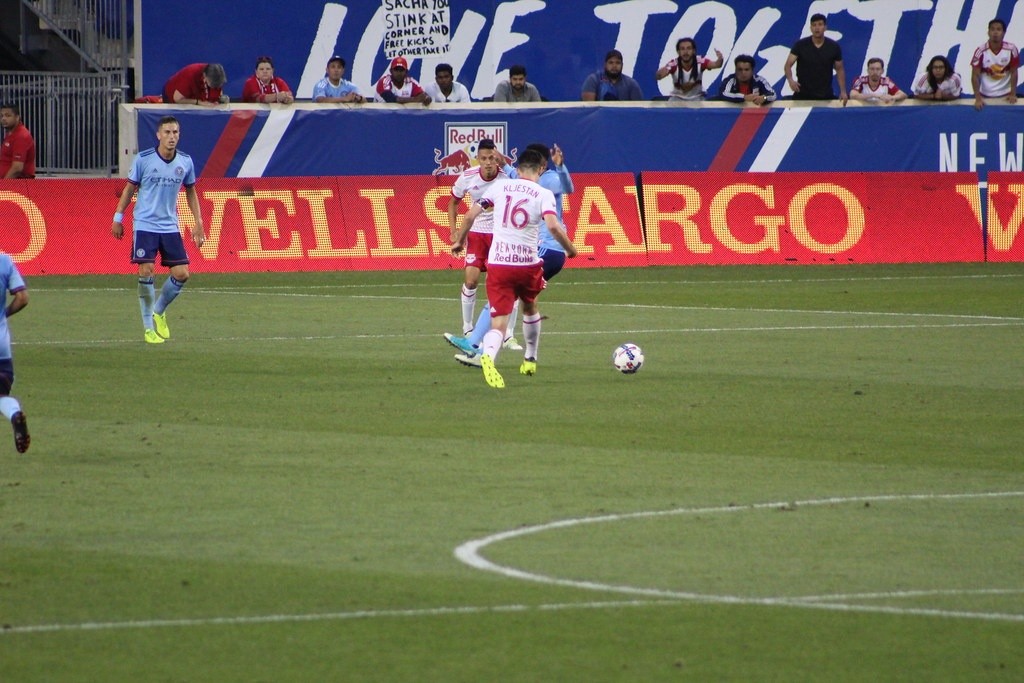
[501,337,523,352]
[520,358,536,374]
[464,330,473,338]
[453,348,482,369]
[153,310,170,338]
[482,354,504,389]
[12,412,31,454]
[443,332,477,358]
[144,329,164,344]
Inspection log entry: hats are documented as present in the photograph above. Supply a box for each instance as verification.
[391,57,407,70]
[327,55,346,68]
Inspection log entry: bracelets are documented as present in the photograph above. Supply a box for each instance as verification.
[359,96,363,102]
[113,212,123,222]
[763,94,767,102]
[196,99,199,106]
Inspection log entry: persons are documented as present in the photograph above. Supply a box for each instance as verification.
[849,58,908,103]
[162,62,229,107]
[425,64,471,103]
[912,55,961,100]
[110,115,206,343]
[443,139,576,388]
[493,65,541,102]
[581,50,642,101]
[656,38,724,101]
[312,56,367,104]
[373,57,431,106]
[0,254,30,453]
[0,104,36,179]
[971,19,1019,110]
[719,55,777,105]
[242,56,294,105]
[784,14,849,107]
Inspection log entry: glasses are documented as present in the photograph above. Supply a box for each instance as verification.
[932,64,945,69]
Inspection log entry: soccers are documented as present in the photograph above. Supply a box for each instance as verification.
[612,343,644,374]
